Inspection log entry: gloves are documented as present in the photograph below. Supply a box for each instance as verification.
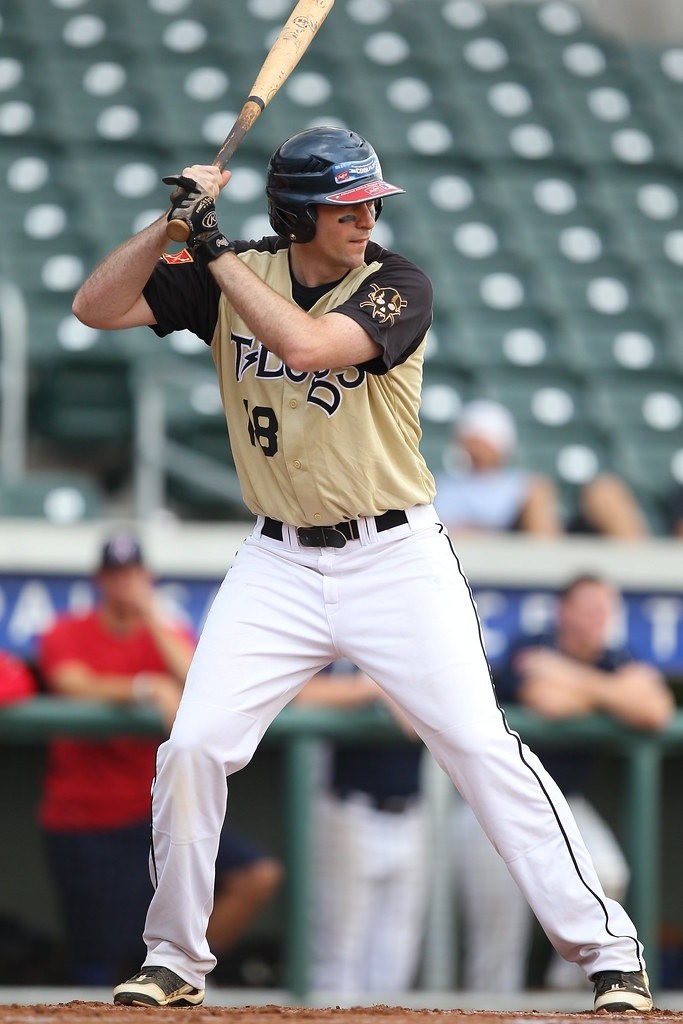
[160,175,234,265]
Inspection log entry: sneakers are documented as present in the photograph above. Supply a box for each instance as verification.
[592,961,652,1013]
[114,965,206,1009]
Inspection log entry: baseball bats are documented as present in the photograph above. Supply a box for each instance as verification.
[164,0,336,243]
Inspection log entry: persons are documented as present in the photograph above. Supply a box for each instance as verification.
[456,578,674,994]
[285,631,435,993]
[415,397,554,535]
[73,128,660,1016]
[41,530,280,989]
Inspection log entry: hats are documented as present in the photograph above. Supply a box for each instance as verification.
[93,534,144,575]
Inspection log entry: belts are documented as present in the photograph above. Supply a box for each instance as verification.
[261,510,413,547]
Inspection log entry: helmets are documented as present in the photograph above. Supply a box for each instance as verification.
[265,126,408,243]
